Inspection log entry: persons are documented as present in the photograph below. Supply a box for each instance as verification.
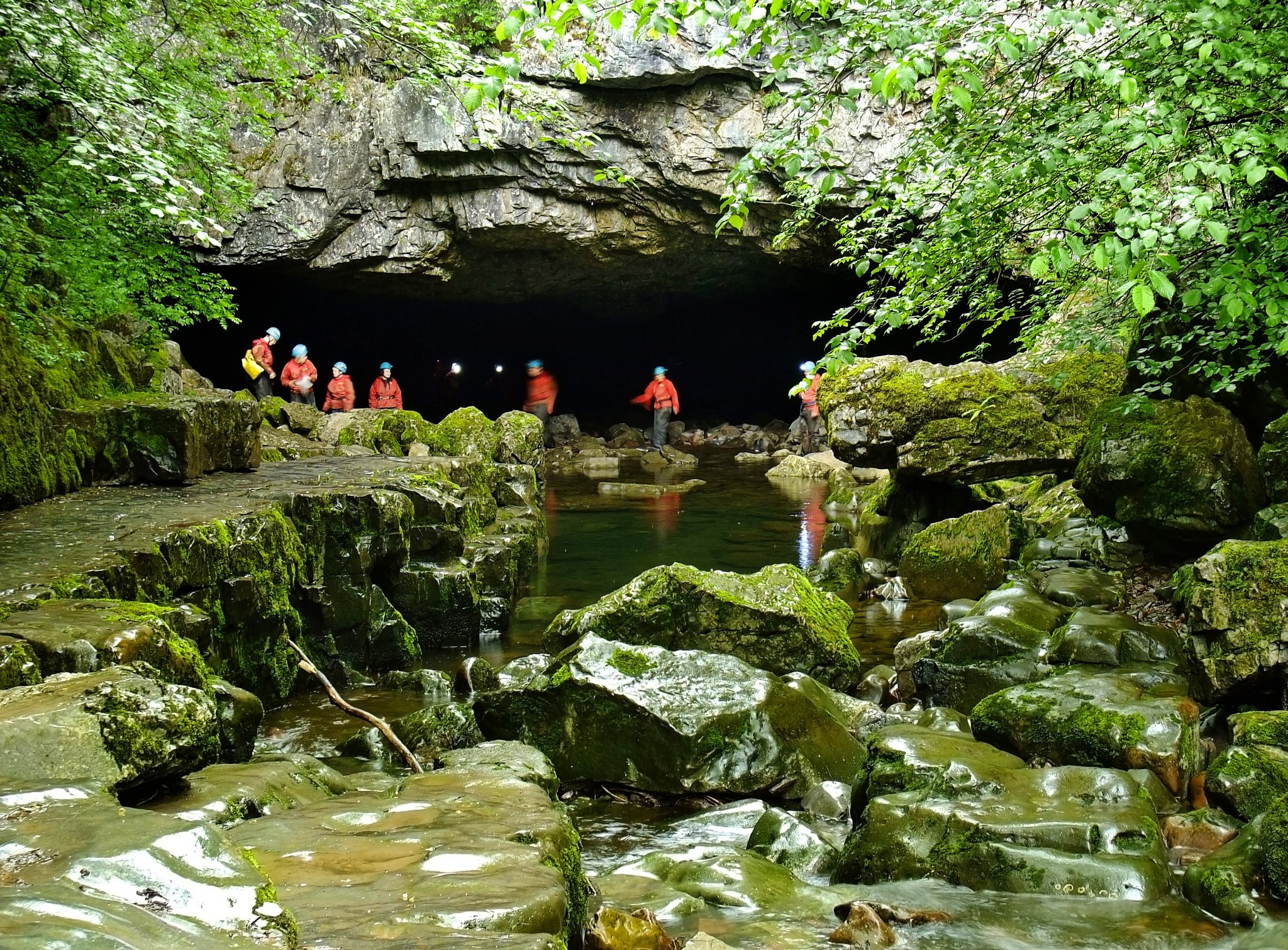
[522,359,558,450]
[369,363,403,410]
[252,327,280,400]
[632,366,679,449]
[787,361,822,453]
[281,344,318,406]
[322,362,356,414]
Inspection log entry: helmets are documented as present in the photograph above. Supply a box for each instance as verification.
[380,362,391,370]
[528,359,543,367]
[800,361,816,370]
[334,362,347,373]
[266,327,280,340]
[291,344,308,356]
[653,366,666,375]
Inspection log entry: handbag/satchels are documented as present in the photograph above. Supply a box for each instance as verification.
[241,349,264,380]
